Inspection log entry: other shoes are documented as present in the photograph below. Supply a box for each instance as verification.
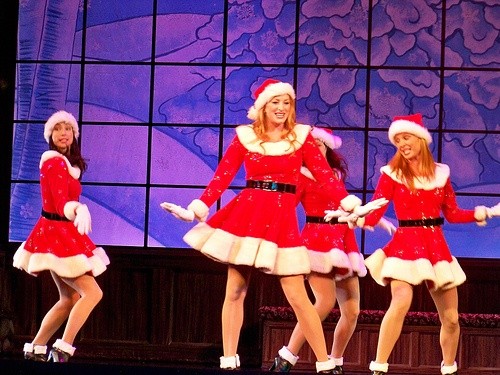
[219,354,241,371]
[370,361,389,375]
[314,360,332,374]
[441,360,458,375]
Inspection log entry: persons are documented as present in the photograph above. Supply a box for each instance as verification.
[13,111,110,363]
[160,77,500,375]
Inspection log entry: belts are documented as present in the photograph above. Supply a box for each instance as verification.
[41,207,70,221]
[246,180,296,194]
[306,216,347,224]
[398,217,444,227]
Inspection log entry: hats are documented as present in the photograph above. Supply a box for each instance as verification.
[43,110,79,144]
[310,127,343,150]
[246,79,296,120]
[387,113,432,146]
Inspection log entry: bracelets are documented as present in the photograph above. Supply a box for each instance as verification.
[74,205,78,216]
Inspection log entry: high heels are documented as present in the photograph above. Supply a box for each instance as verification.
[268,346,299,372]
[47,339,76,362]
[23,343,48,361]
[330,357,344,374]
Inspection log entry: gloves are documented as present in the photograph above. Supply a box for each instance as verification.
[379,218,397,235]
[353,197,389,218]
[74,204,92,235]
[324,210,350,222]
[160,202,195,222]
[487,202,500,219]
